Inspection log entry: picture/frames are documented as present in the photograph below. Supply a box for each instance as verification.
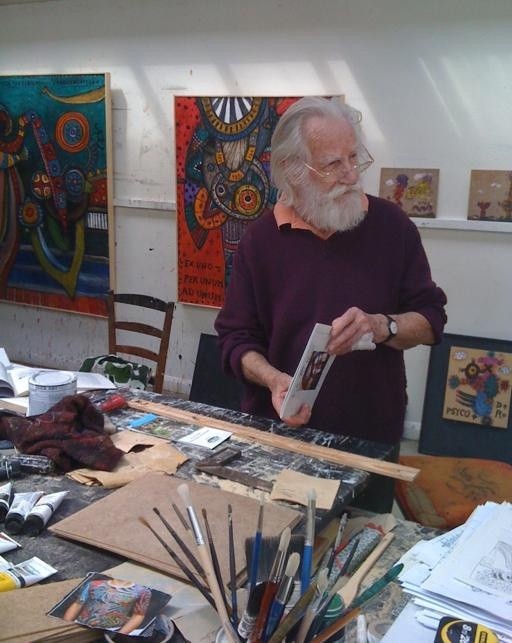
[0,70,116,319]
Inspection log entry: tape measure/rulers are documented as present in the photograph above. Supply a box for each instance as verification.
[127,397,422,483]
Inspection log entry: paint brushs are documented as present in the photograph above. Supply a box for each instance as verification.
[139,483,403,643]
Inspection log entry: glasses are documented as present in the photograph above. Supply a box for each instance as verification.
[299,142,375,184]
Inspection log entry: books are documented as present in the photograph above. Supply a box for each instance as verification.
[0,347,116,415]
[278,323,337,419]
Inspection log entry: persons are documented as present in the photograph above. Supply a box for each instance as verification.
[214,96,447,514]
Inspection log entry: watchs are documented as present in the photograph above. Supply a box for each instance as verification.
[380,311,398,344]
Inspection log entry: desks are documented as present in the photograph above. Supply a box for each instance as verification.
[0,382,447,643]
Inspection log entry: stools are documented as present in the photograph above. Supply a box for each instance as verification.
[389,454,512,532]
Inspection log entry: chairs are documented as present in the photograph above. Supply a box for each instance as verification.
[105,287,176,393]
